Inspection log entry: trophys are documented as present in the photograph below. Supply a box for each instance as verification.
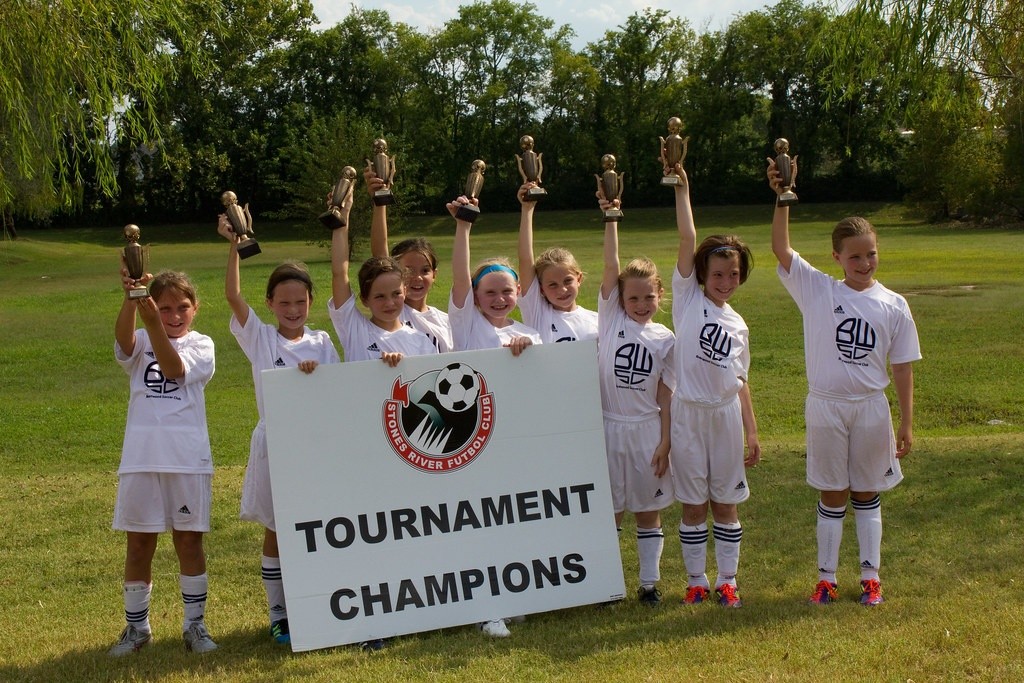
[595,154,625,223]
[119,224,150,301]
[317,165,357,230]
[364,139,397,207]
[659,117,690,188]
[514,135,549,202]
[454,159,487,224]
[774,137,799,206]
[218,191,262,260]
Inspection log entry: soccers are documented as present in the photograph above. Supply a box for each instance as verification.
[435,363,480,413]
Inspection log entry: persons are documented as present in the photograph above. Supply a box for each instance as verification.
[445,195,544,638]
[661,161,760,607]
[766,152,925,608]
[213,216,340,645]
[590,191,678,605]
[324,169,456,367]
[517,181,599,346]
[106,267,216,661]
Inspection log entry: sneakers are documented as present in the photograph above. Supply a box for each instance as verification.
[270,618,290,644]
[859,577,883,606]
[683,586,711,604]
[182,622,218,654]
[636,584,662,606]
[483,618,511,637]
[808,580,838,603]
[106,625,152,658]
[714,583,742,608]
